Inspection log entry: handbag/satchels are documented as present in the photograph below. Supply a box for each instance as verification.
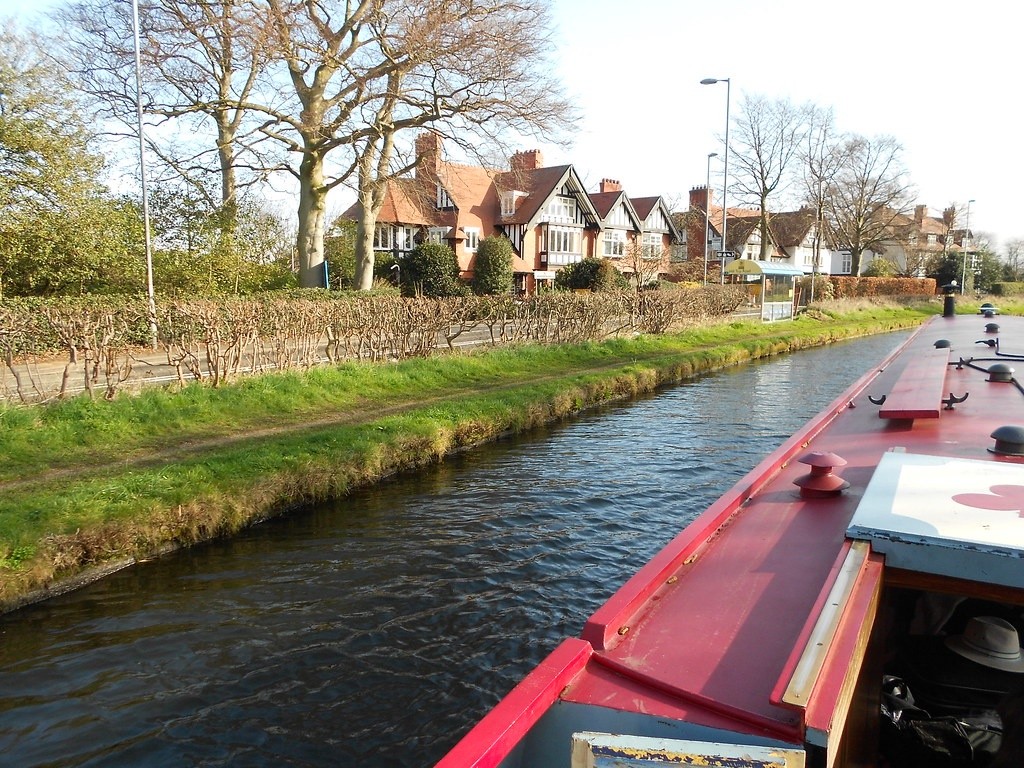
[880,675,1004,765]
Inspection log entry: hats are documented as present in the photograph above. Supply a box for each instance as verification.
[944,616,1024,673]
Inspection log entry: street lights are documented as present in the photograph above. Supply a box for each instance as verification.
[959,199,977,296]
[699,76,731,287]
[702,152,719,285]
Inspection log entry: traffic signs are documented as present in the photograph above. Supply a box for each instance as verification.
[717,251,736,258]
